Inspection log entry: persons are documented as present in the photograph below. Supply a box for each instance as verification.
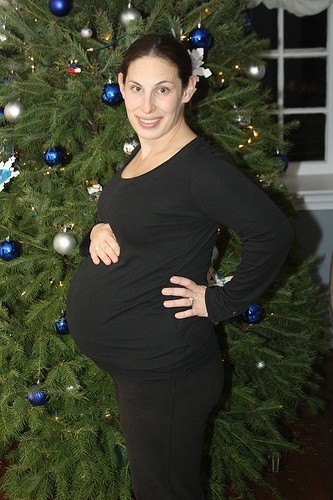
[64,32,297,500]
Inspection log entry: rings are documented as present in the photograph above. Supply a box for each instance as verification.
[189,297,194,307]
[94,246,101,252]
